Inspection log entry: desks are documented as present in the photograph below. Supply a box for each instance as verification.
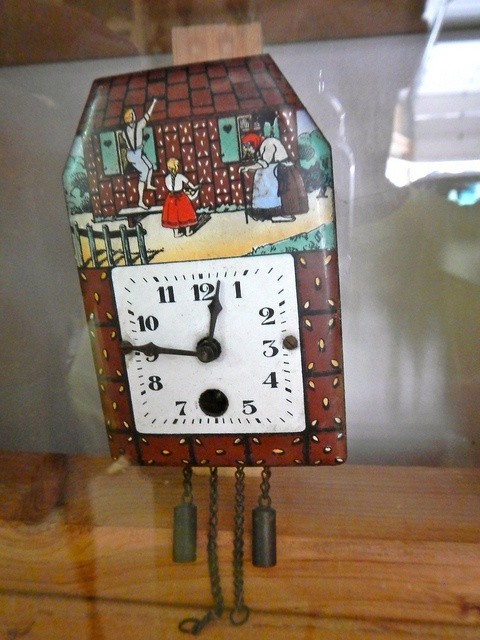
[0,444,479,640]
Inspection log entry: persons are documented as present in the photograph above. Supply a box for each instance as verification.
[117,97,158,209]
[161,156,199,237]
[238,131,309,222]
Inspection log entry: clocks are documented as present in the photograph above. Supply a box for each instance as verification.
[62,54,348,637]
